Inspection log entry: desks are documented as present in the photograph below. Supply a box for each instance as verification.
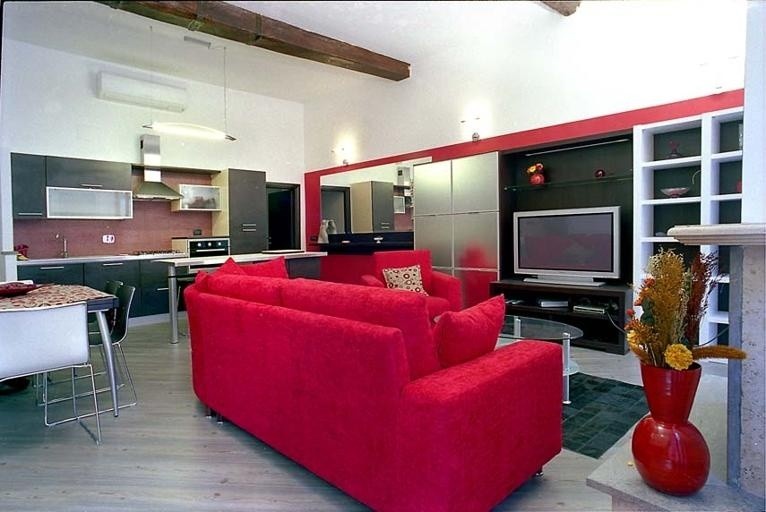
[0,282,120,417]
[588,376,766,512]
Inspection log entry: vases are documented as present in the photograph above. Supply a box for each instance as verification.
[528,173,545,185]
[631,360,713,498]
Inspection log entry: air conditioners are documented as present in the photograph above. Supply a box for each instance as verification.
[96,72,188,113]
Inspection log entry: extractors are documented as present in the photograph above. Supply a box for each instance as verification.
[132,134,185,202]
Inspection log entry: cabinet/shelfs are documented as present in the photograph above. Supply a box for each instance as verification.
[169,183,222,213]
[17,264,84,286]
[84,260,141,318]
[632,106,746,365]
[45,155,134,220]
[351,181,393,234]
[210,168,269,255]
[140,256,188,316]
[11,153,46,220]
[488,277,634,358]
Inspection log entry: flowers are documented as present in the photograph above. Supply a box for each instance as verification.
[625,244,748,372]
[525,161,545,175]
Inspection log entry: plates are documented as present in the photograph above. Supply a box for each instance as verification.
[0,284,46,296]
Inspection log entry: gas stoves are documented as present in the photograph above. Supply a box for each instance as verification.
[121,249,187,260]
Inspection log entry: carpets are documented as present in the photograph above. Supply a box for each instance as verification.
[562,371,651,460]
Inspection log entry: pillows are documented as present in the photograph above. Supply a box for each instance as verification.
[380,263,429,297]
[238,253,290,279]
[217,257,246,275]
[434,292,505,369]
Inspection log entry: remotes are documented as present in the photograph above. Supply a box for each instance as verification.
[512,300,522,304]
[505,300,514,303]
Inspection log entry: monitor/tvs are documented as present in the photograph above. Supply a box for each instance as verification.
[513,206,621,287]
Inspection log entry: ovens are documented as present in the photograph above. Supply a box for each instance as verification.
[172,238,230,275]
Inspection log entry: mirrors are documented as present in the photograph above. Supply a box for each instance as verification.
[320,156,432,233]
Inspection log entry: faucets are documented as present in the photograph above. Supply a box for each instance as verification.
[62,238,69,258]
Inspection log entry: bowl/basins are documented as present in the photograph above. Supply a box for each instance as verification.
[661,187,691,198]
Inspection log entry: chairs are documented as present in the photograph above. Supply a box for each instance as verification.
[43,283,138,429]
[31,278,122,407]
[0,301,102,447]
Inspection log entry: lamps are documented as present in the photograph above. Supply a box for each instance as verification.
[330,148,348,166]
[460,117,481,142]
[142,26,236,142]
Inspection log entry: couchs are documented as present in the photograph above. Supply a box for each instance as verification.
[182,281,565,512]
[361,248,464,330]
